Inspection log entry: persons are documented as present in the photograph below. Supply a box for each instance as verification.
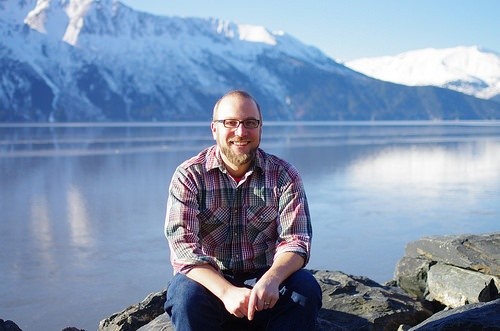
[163,90,323,331]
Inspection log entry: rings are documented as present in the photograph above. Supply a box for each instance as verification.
[264,302,270,306]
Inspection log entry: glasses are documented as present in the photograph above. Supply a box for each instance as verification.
[214,119,260,129]
